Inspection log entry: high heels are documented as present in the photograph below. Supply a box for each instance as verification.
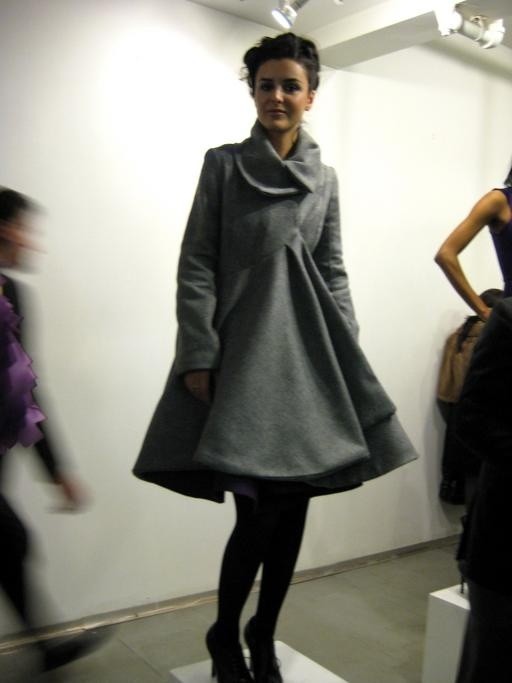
[206,622,254,683]
[244,616,283,683]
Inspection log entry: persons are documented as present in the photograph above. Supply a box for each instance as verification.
[434,165,512,323]
[438,298,512,683]
[131,34,420,683]
[435,289,506,506]
[0,186,116,673]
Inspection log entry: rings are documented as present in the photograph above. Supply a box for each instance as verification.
[192,385,200,392]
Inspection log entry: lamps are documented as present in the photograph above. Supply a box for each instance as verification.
[269,0,311,32]
[431,0,509,50]
